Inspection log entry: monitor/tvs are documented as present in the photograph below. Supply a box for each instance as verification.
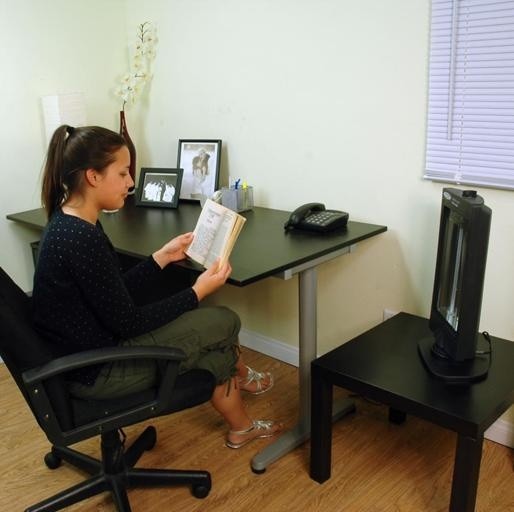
[417,188,492,384]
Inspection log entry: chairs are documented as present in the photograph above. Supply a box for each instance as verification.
[0,268,215,512]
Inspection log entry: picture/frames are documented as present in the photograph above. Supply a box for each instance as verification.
[134,168,183,208]
[177,139,222,204]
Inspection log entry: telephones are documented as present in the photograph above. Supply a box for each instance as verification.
[283,202,349,235]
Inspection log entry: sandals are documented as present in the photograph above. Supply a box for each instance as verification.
[238,365,275,397]
[225,418,285,450]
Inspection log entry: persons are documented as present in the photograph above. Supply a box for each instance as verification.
[191,149,211,199]
[30,124,283,448]
[143,180,176,202]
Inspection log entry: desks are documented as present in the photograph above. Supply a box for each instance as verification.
[8,206,388,474]
[310,311,514,512]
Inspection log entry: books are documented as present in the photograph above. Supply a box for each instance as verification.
[183,199,247,274]
